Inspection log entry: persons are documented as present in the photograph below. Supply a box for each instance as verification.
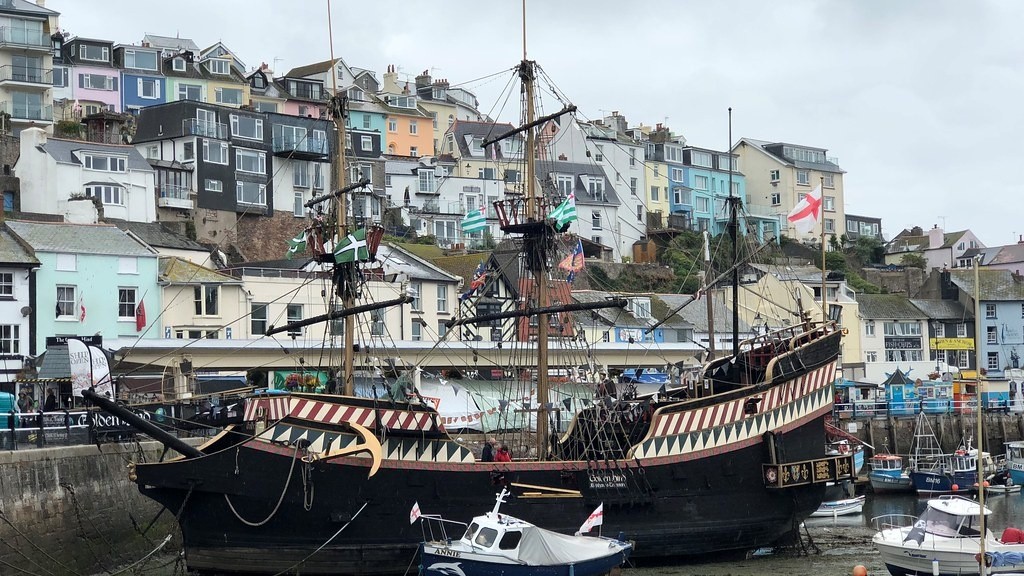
[42,388,55,413]
[482,438,511,462]
[13,390,34,428]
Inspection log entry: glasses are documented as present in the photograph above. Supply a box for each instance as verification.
[502,449,506,451]
[491,443,495,446]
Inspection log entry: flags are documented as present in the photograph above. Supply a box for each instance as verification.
[558,238,585,273]
[410,501,421,525]
[334,228,370,264]
[460,205,487,234]
[136,300,147,331]
[579,503,603,533]
[284,228,308,261]
[546,190,577,230]
[461,260,486,301]
[786,183,823,235]
[81,304,85,321]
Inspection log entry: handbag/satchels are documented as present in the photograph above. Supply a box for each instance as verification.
[27,397,37,413]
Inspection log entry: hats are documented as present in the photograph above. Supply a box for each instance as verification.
[457,438,463,444]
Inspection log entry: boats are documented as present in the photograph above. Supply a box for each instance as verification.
[906,399,1024,493]
[809,494,867,517]
[824,439,866,476]
[417,486,636,576]
[71,0,850,576]
[871,495,1024,576]
[865,453,913,494]
